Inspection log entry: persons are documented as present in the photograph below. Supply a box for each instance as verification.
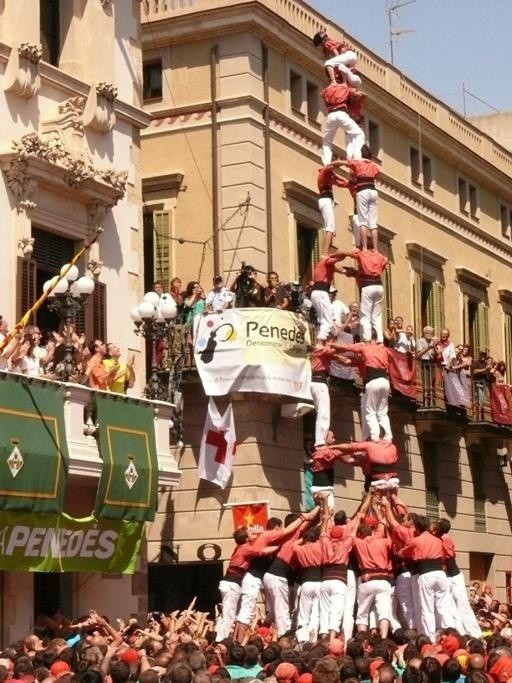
[150,267,300,369]
[383,316,512,415]
[214,488,482,645]
[303,243,399,528]
[0,581,511,683]
[0,315,139,394]
[311,30,391,267]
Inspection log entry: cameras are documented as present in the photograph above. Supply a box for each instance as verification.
[237,262,252,286]
[153,611,160,615]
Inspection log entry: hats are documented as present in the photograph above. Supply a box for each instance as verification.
[442,636,458,654]
[51,662,69,675]
[275,662,313,683]
[367,517,378,528]
[122,649,137,664]
[328,639,344,657]
[331,527,342,539]
